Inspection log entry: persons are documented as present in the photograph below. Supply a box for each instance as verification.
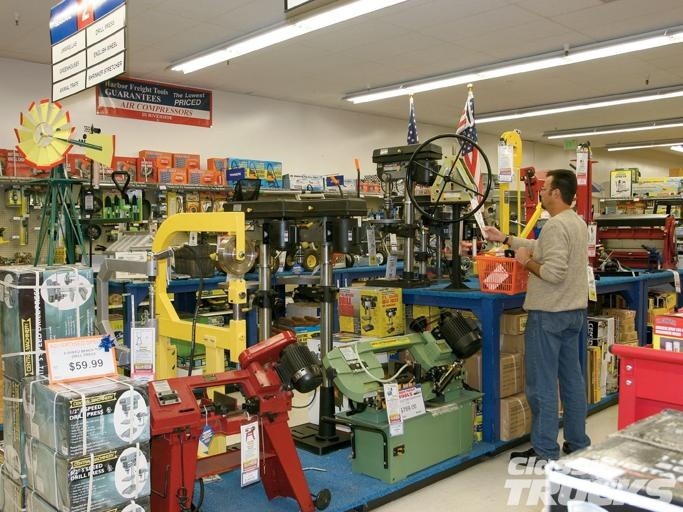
[481,168,591,470]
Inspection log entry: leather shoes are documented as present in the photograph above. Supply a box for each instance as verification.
[511,449,552,469]
[563,443,575,455]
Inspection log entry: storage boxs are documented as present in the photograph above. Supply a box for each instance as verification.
[227,158,282,189]
[23,373,151,457]
[499,312,528,334]
[66,154,91,179]
[475,256,529,295]
[139,150,172,169]
[501,393,531,441]
[0,376,27,485]
[0,149,50,178]
[282,173,323,191]
[0,265,94,383]
[100,157,137,183]
[338,286,404,337]
[173,154,200,169]
[158,168,187,184]
[0,468,24,512]
[651,312,683,353]
[189,169,216,185]
[137,158,158,182]
[502,335,525,353]
[25,436,151,512]
[323,175,344,191]
[207,158,228,171]
[462,353,522,398]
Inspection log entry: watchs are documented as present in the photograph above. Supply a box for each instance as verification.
[503,234,509,245]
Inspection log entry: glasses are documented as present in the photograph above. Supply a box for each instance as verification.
[541,186,553,191]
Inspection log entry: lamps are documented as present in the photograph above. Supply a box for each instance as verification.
[542,117,683,141]
[605,138,683,151]
[164,0,408,74]
[474,84,683,125]
[341,25,682,106]
